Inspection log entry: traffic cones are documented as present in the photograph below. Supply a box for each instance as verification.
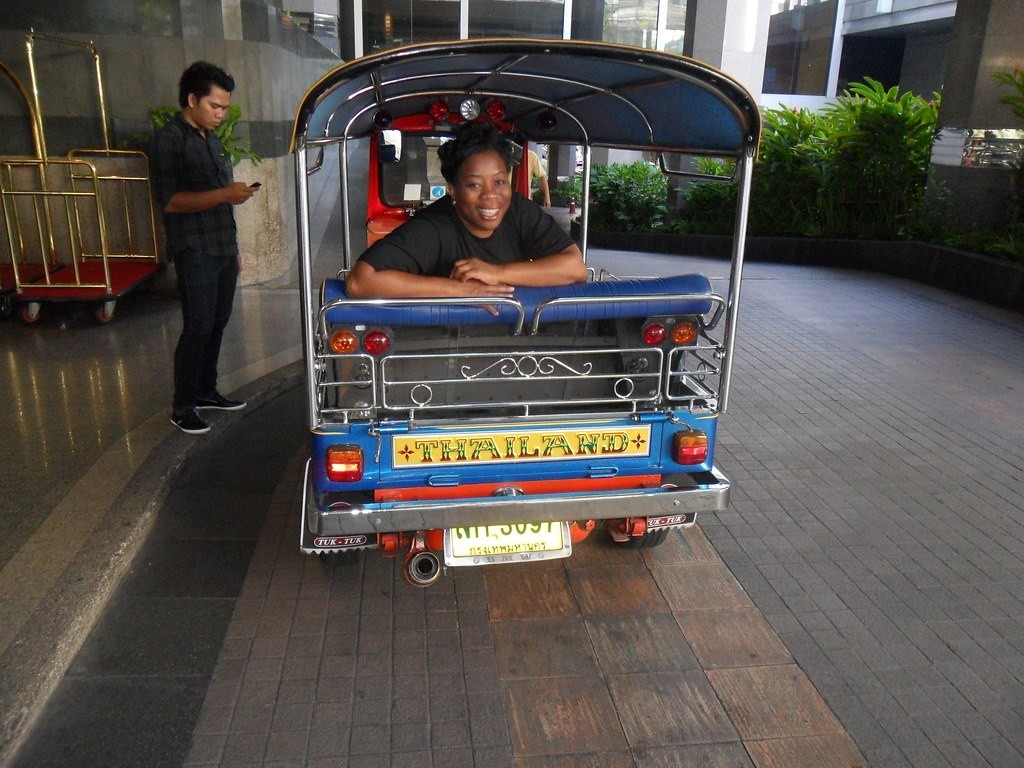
[568,194,576,214]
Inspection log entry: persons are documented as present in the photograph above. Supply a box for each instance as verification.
[345,122,588,317]
[508,150,551,208]
[151,61,261,434]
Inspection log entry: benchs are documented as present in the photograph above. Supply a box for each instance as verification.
[323,273,713,410]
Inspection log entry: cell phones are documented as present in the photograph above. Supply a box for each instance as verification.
[250,181,262,187]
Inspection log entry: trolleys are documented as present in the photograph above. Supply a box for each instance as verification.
[0,27,167,327]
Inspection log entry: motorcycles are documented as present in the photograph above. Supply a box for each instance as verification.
[285,30,764,590]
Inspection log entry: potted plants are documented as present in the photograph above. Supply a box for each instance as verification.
[116,105,263,296]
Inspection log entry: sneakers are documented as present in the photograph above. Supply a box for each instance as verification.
[195,388,247,411]
[171,408,211,434]
